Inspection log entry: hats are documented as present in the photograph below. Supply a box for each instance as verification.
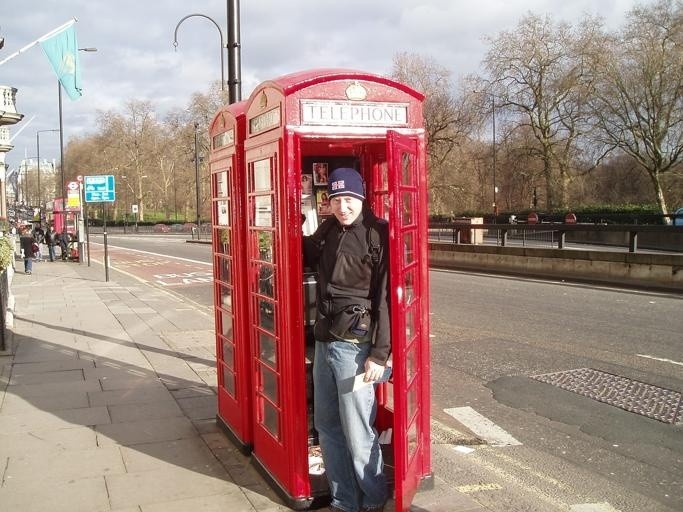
[325,168,367,201]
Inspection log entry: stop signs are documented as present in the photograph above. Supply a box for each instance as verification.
[565,214,576,224]
[527,212,538,224]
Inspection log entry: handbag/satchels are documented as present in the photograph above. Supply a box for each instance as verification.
[32,244,38,253]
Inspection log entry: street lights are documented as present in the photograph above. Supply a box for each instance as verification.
[58,48,98,210]
[192,122,202,240]
[37,128,60,228]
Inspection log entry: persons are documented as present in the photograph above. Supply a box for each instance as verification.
[15,222,69,276]
[299,167,392,512]
[301,176,310,195]
[318,166,327,183]
[321,192,330,208]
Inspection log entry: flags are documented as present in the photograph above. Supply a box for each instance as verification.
[37,21,83,102]
